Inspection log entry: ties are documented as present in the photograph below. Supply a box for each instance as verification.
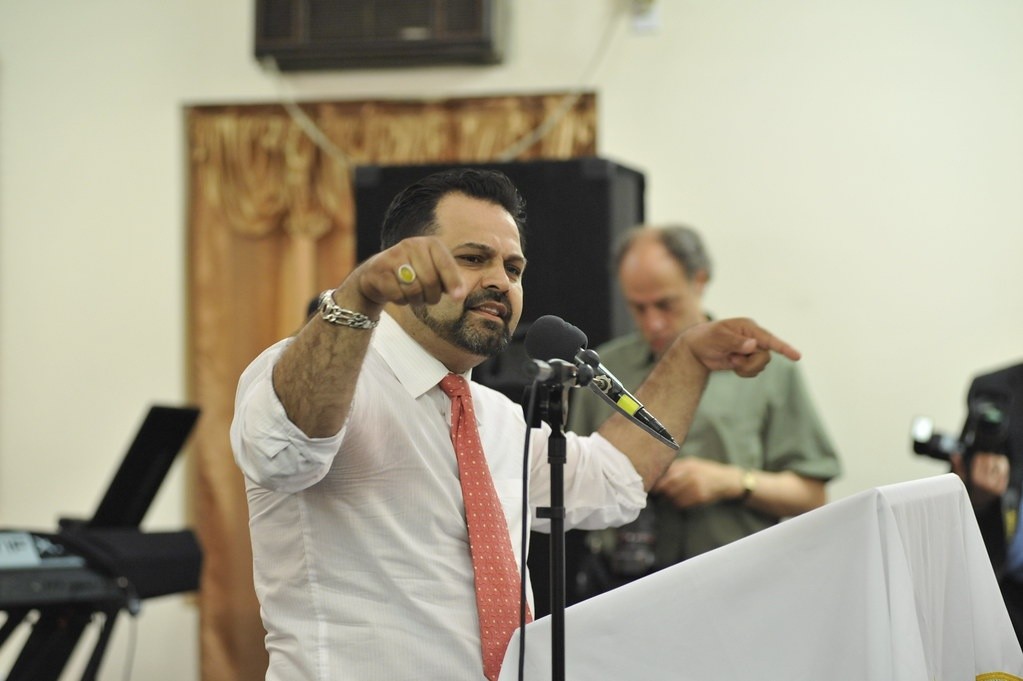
[439,374,533,681]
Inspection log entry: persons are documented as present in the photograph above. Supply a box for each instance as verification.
[570,224,843,599]
[231,169,800,681]
[950,362,1023,653]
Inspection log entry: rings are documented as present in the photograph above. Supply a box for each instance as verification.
[398,265,417,283]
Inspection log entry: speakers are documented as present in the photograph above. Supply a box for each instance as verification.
[347,156,645,418]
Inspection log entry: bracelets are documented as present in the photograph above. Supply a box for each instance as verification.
[318,289,381,329]
[733,465,753,504]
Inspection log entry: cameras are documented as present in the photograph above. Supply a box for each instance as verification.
[913,400,1004,465]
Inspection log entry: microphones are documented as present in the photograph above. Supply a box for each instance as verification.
[526,315,675,444]
